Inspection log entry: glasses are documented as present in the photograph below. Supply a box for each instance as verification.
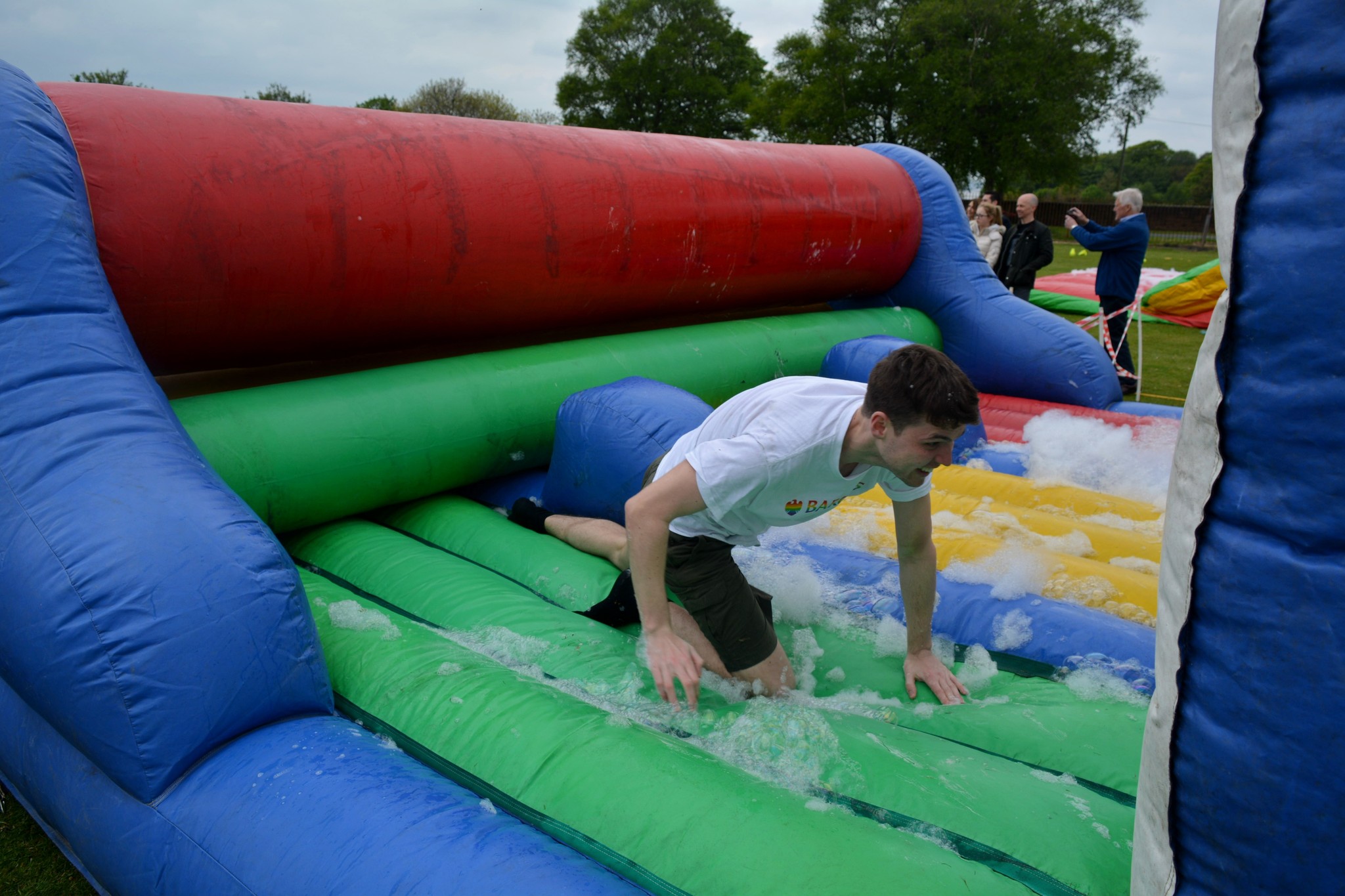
[974,214,988,219]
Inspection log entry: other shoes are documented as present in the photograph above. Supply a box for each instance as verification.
[1122,385,1136,394]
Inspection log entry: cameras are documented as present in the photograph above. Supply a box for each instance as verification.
[1066,210,1074,217]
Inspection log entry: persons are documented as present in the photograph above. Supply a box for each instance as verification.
[967,191,1010,229]
[509,345,980,711]
[1065,188,1149,396]
[969,203,1006,270]
[993,193,1054,302]
